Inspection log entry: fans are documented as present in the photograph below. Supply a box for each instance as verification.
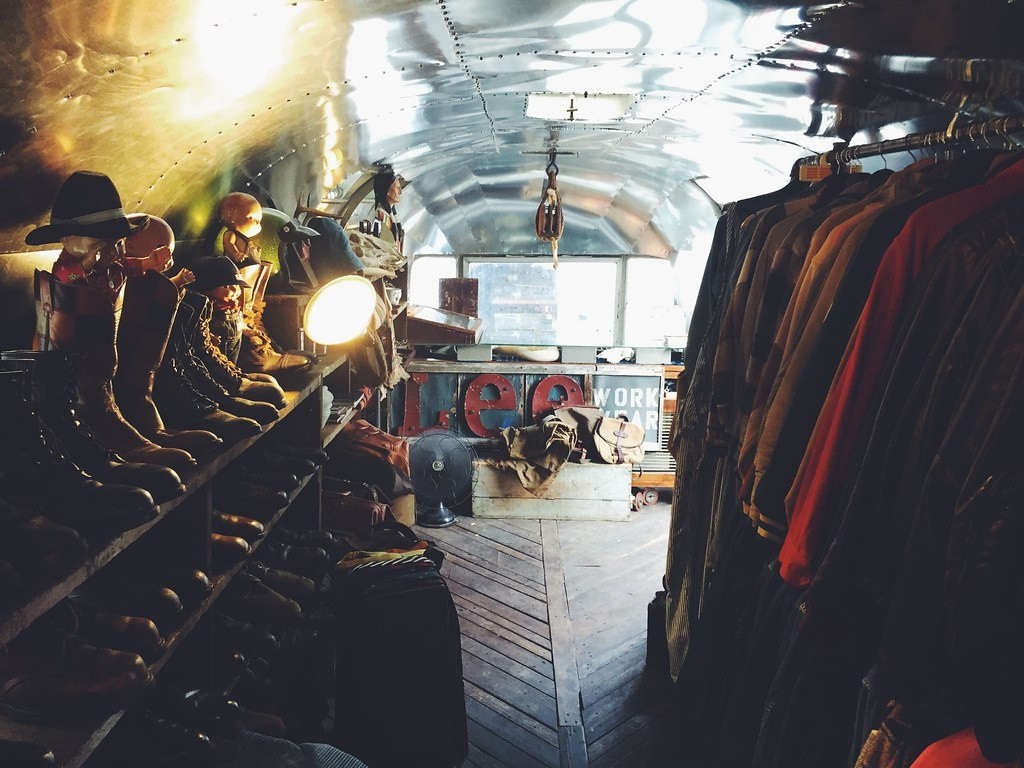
[394,425,481,529]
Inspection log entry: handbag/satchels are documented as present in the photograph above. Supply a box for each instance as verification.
[321,419,417,536]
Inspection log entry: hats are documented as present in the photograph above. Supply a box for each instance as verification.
[26,171,150,246]
[306,218,358,274]
[192,255,250,292]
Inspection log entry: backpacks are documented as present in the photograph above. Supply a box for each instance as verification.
[594,413,646,464]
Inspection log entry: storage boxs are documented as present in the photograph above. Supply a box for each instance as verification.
[471,455,633,522]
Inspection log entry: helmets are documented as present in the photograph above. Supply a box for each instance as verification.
[125,214,176,271]
[214,210,321,292]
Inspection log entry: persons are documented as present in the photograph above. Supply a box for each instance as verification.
[373,167,403,251]
[125,214,196,294]
[187,256,261,366]
[24,170,149,302]
[218,192,263,268]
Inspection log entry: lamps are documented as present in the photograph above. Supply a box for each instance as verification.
[260,217,378,346]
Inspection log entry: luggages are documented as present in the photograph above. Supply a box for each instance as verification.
[320,560,468,768]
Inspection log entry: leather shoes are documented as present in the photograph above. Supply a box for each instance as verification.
[1,442,336,768]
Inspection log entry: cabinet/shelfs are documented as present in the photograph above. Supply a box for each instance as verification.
[632,365,684,507]
[0,254,411,768]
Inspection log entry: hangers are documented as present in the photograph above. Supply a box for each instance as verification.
[789,58,1024,195]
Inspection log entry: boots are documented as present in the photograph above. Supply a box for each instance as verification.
[0,265,316,604]
